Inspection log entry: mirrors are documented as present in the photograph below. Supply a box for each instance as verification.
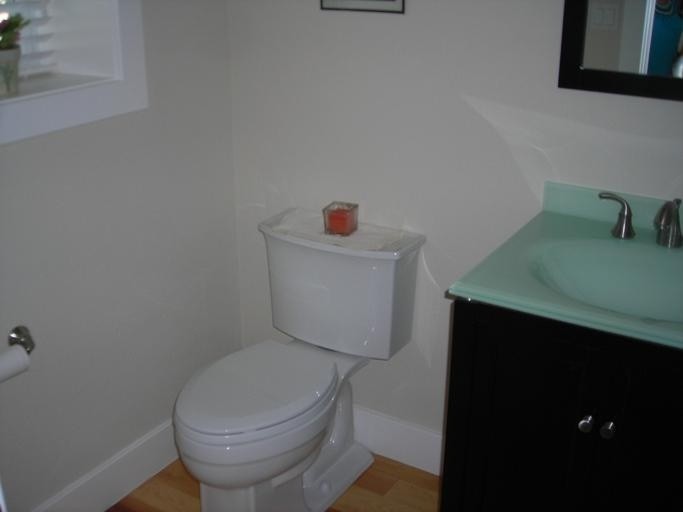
[557,0,683,103]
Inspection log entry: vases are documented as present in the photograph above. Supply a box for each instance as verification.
[1,46,21,99]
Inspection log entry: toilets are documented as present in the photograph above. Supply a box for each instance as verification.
[169,206,425,510]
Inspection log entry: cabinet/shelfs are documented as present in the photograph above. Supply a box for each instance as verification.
[435,302,682,510]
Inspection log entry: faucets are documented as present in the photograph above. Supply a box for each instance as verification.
[655,196,683,246]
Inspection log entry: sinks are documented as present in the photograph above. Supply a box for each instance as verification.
[526,237,683,324]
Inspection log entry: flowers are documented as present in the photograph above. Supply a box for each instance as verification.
[0,12,31,48]
